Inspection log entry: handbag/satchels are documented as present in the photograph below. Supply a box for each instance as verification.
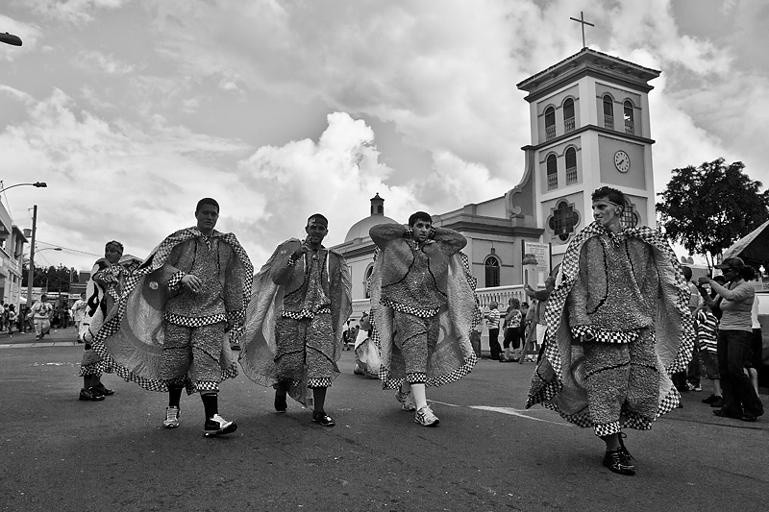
[711,293,724,322]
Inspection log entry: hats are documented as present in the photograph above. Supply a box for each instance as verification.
[712,256,746,273]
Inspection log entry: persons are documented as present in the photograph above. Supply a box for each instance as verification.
[90,196,255,436]
[669,253,769,423]
[0,289,86,341]
[524,185,701,474]
[342,294,540,361]
[77,240,141,400]
[523,276,556,350]
[353,213,483,427]
[234,214,354,427]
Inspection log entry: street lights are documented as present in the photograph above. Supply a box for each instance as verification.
[0,181,47,195]
[26,205,62,310]
[0,32,21,48]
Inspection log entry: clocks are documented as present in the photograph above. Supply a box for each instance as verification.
[614,150,630,173]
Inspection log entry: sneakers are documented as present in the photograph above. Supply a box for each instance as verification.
[310,409,336,427]
[741,405,765,423]
[9,331,50,342]
[674,380,703,392]
[702,393,715,405]
[273,390,287,413]
[603,432,636,476]
[78,386,105,402]
[710,392,725,407]
[163,405,182,428]
[712,406,744,419]
[92,382,116,397]
[394,386,416,411]
[413,406,440,427]
[202,413,238,439]
